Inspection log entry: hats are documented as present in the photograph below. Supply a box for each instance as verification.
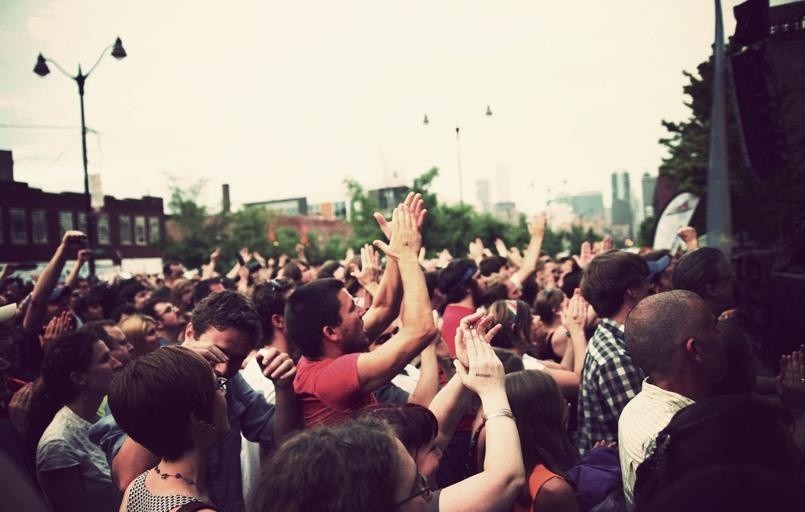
[646,255,671,278]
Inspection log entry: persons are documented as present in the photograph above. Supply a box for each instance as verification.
[1,191,803,511]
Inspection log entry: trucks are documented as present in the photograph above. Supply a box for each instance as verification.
[9,256,164,287]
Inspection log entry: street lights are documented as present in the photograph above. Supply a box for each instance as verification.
[418,103,495,237]
[32,32,128,271]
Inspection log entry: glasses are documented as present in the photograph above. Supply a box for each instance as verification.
[215,378,229,392]
[393,475,434,512]
